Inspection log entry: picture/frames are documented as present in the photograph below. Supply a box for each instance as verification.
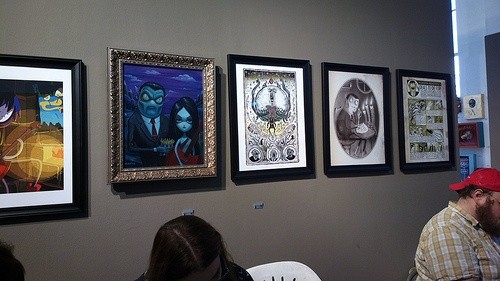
[395,69,455,172]
[106,46,217,184]
[227,53,317,182]
[320,61,392,175]
[0,54,91,225]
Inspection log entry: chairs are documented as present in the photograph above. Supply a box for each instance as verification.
[245,260,323,281]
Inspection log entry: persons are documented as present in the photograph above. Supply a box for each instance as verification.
[415,170,500,281]
[133,215,256,281]
[0,239,26,281]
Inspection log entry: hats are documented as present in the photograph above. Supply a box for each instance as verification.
[449,169,500,192]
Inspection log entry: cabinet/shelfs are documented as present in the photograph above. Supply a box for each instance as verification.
[458,122,484,148]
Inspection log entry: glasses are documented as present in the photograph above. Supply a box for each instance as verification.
[216,254,229,281]
